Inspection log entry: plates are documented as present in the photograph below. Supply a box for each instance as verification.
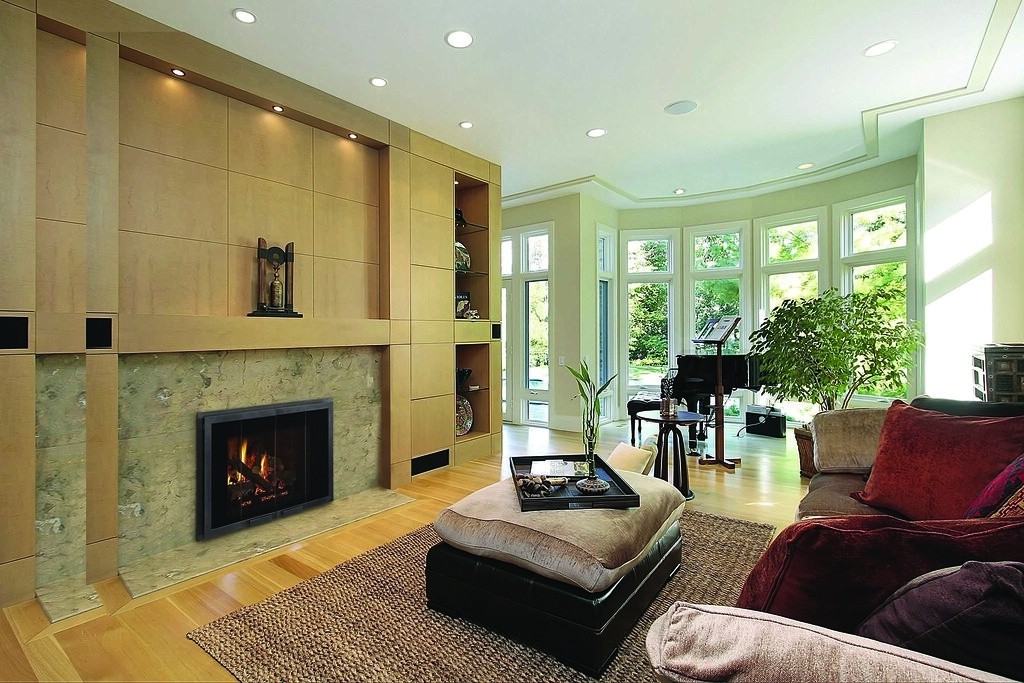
[455,241,470,270]
[456,395,473,436]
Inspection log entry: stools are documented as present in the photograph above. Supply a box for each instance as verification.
[426,469,687,680]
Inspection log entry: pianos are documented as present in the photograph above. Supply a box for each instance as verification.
[660,353,763,470]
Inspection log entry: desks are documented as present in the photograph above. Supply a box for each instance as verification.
[636,410,705,501]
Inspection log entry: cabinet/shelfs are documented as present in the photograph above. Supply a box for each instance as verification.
[453,170,490,321]
[454,342,491,445]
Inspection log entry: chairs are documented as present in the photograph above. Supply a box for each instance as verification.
[607,436,658,475]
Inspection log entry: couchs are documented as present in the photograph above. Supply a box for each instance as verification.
[646,407,1024,683]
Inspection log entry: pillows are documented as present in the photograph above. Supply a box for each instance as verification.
[850,394,1024,521]
[607,442,652,473]
[735,516,1024,682]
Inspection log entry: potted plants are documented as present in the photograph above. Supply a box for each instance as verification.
[564,360,620,494]
[745,283,925,479]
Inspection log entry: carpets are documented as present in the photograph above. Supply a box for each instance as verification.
[186,510,776,683]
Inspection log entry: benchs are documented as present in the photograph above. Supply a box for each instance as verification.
[627,391,663,447]
